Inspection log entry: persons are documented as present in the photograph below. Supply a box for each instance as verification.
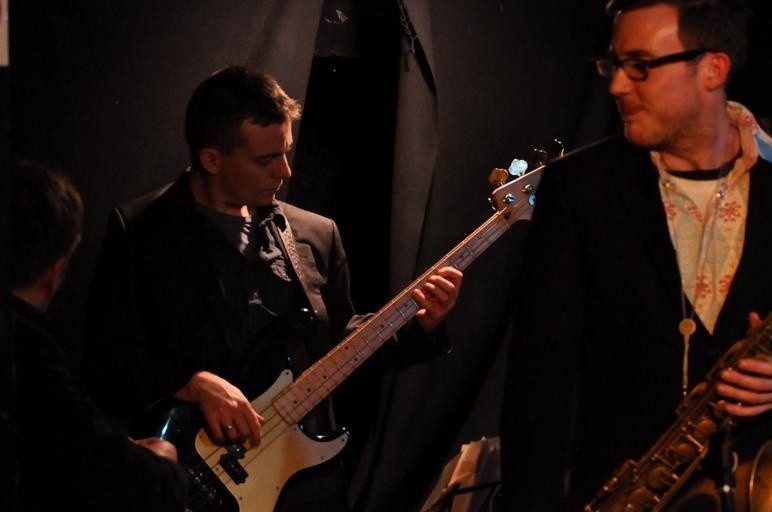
[87,65,464,509]
[500,0,770,512]
[1,156,190,509]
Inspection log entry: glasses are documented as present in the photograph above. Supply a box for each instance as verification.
[595,46,703,83]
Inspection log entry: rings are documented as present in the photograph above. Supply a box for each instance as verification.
[225,422,236,432]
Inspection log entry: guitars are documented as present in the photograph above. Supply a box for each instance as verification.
[126,136,567,512]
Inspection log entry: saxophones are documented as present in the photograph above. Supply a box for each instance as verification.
[582,313,772,512]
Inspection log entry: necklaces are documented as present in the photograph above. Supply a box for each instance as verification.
[663,125,735,386]
